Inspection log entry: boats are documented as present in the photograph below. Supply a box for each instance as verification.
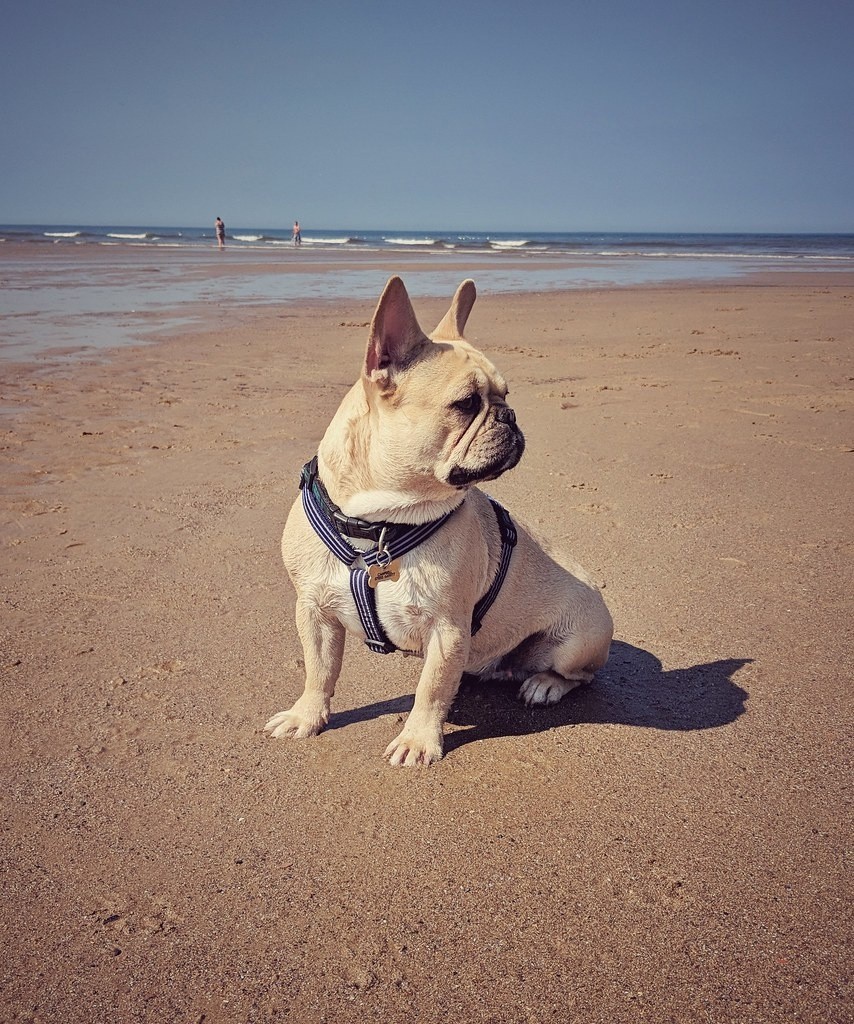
[42,230,81,238]
[384,238,441,245]
[488,239,532,247]
[232,234,264,241]
[106,231,153,239]
[300,237,358,243]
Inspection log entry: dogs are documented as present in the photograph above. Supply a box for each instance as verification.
[261,275,616,770]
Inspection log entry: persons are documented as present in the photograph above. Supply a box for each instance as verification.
[216,217,225,246]
[294,222,300,247]
